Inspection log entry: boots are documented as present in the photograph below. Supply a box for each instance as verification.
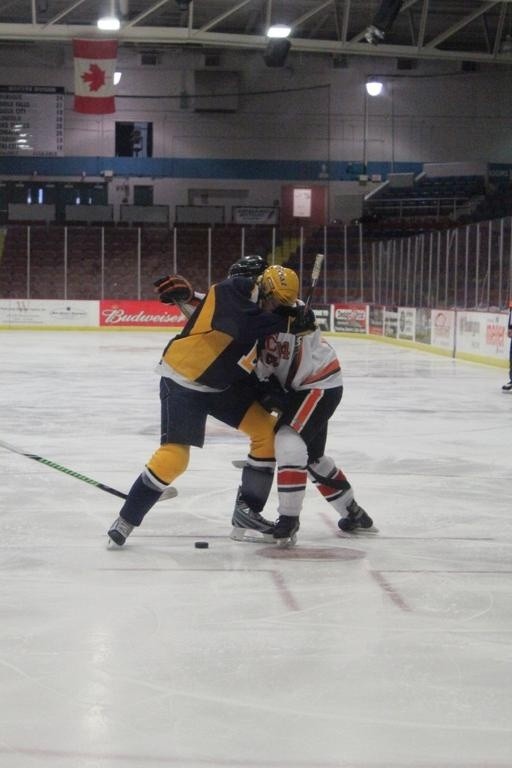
[107,515,131,546]
[232,486,279,532]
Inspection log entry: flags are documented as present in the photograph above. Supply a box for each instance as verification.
[71,39,118,115]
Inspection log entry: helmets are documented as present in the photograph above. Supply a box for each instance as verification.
[261,266,300,307]
[227,254,267,280]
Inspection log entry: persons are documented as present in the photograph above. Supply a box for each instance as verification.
[155,262,374,540]
[105,250,319,546]
[501,290,512,390]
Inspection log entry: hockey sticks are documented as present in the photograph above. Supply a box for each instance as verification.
[0,441,178,501]
[232,253,324,469]
[174,299,350,490]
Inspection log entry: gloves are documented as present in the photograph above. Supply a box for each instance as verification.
[261,383,294,411]
[290,304,317,335]
[154,273,194,306]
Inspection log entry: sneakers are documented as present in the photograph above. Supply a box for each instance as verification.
[273,514,301,538]
[337,500,372,531]
[502,380,512,390]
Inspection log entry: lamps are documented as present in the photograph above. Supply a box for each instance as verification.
[365,76,383,96]
[267,23,291,38]
